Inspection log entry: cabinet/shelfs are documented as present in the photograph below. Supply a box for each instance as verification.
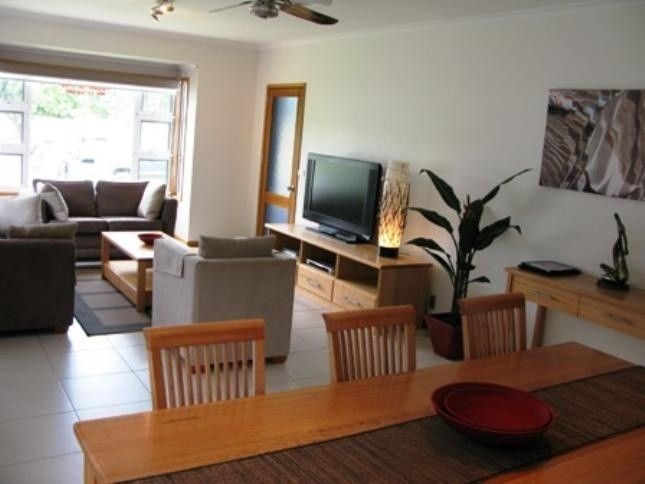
[264,220,435,328]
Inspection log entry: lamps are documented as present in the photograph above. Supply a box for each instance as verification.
[376,158,410,259]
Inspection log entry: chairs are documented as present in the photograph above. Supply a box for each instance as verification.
[320,302,417,382]
[142,318,268,411]
[458,292,529,364]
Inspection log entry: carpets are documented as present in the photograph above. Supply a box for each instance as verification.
[73,258,154,340]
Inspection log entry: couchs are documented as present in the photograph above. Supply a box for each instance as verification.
[150,255,299,374]
[0,193,76,340]
[31,180,180,265]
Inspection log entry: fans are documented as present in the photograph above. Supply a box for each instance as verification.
[208,2,338,29]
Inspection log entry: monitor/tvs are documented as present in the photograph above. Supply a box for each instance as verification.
[304,153,382,244]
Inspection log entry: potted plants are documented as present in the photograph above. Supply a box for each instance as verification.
[403,167,532,361]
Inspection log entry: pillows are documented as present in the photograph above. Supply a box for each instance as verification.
[2,199,43,236]
[201,234,275,256]
[32,180,69,222]
[136,178,169,217]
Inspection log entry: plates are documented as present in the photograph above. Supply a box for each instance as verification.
[430,381,556,441]
[137,233,163,245]
[442,385,550,432]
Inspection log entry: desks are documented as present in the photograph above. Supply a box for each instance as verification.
[502,264,645,341]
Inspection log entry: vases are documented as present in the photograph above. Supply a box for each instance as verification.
[427,315,462,361]
